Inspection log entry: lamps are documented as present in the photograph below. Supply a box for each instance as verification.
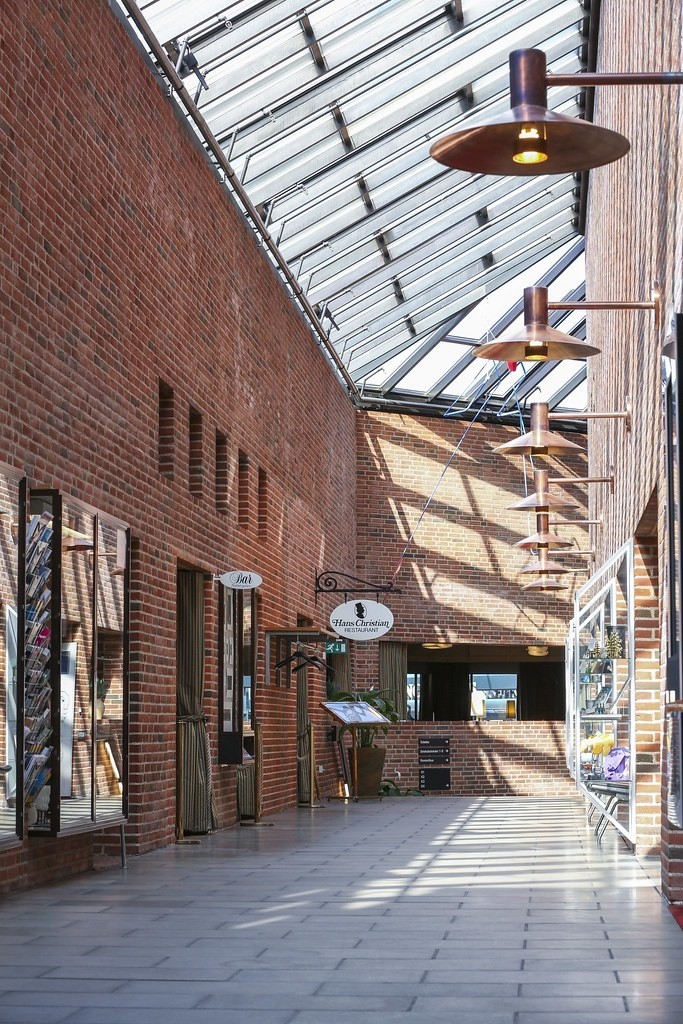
[506,470,615,513]
[521,547,594,574]
[493,402,631,459]
[521,568,589,591]
[431,48,681,174]
[470,285,664,360]
[514,514,602,550]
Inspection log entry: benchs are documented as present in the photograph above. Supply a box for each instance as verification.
[579,777,631,848]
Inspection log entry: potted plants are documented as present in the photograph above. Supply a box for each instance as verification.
[333,686,401,796]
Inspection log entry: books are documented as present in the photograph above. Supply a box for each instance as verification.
[25,511,54,806]
